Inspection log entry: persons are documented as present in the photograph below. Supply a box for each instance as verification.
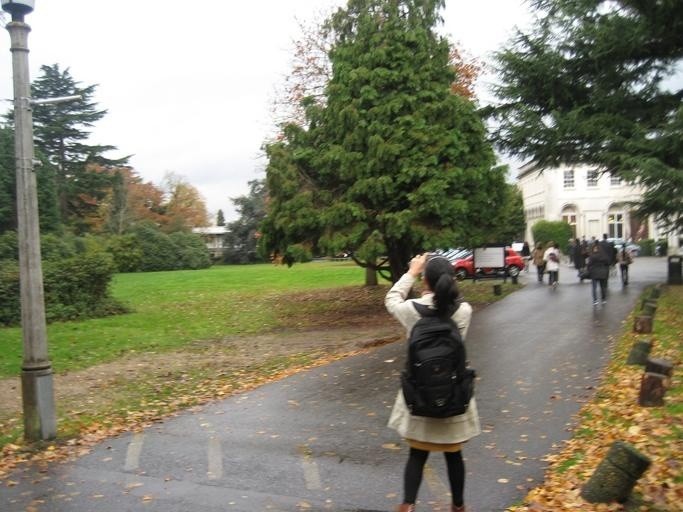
[518,233,635,308]
[382,252,484,511]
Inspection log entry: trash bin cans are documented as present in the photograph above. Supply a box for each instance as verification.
[667,255,683,284]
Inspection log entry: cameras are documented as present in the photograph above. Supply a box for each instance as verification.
[425,254,442,263]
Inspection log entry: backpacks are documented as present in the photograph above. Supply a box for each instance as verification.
[400,300,476,419]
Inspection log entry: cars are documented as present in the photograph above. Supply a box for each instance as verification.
[598,237,641,256]
[421,243,522,284]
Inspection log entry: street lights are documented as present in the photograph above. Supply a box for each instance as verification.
[1,0,56,444]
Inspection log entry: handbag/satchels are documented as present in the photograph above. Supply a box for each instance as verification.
[578,266,589,279]
